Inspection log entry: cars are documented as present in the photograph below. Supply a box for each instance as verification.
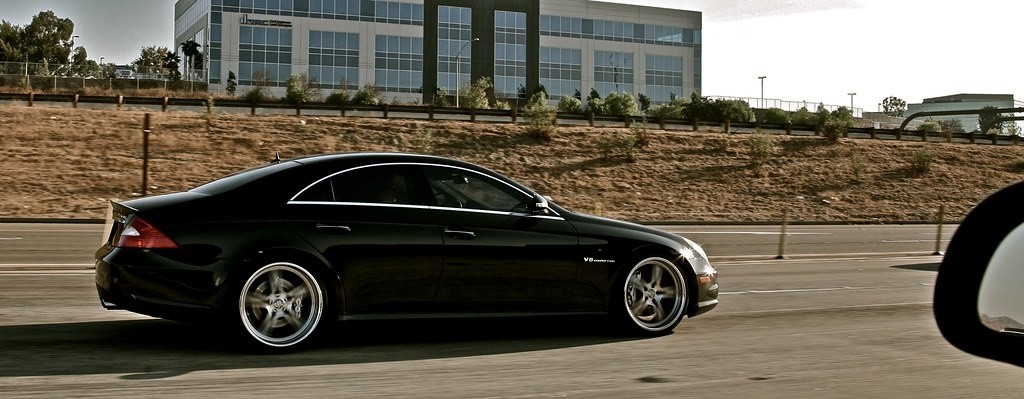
[93,150,720,356]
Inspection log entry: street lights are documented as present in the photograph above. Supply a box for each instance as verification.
[878,103,882,121]
[758,76,767,110]
[71,35,80,76]
[456,36,481,108]
[846,93,857,118]
[99,56,105,78]
